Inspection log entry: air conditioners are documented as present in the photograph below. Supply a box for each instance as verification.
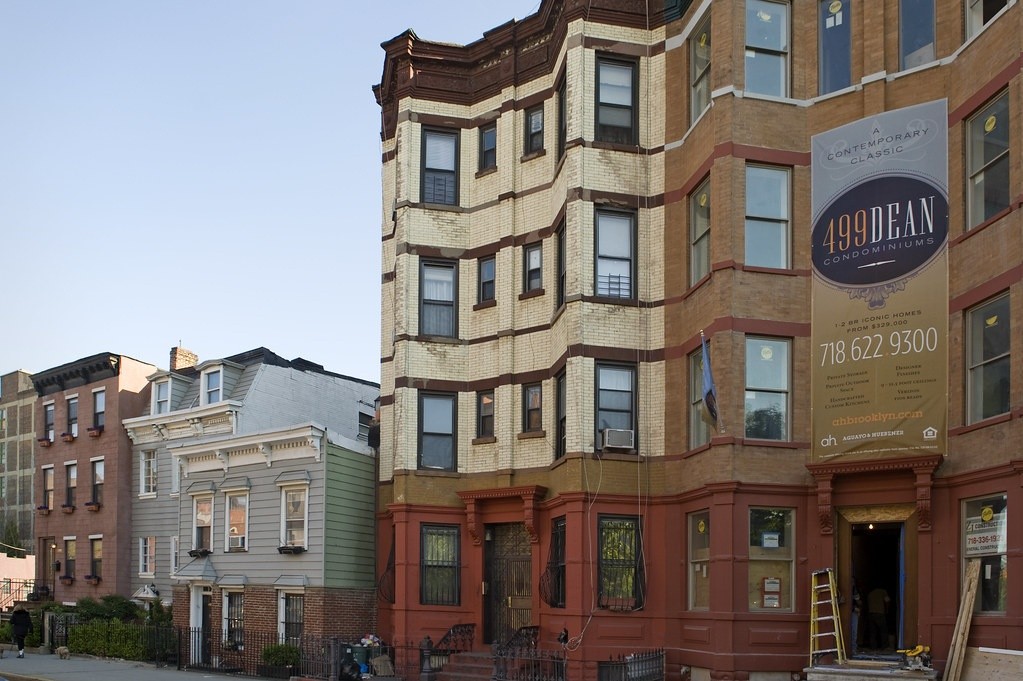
[602,429,634,449]
[230,536,243,547]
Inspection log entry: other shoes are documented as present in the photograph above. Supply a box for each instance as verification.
[16,654,26,658]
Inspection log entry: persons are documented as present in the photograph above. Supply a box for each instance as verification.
[10,604,34,659]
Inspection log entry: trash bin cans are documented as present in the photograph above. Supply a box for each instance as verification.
[347,645,386,675]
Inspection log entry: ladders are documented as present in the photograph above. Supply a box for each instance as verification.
[811,567,847,669]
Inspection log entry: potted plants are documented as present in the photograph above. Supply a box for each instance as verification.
[277,544,303,554]
[257,644,302,679]
[187,548,208,557]
[36,428,100,585]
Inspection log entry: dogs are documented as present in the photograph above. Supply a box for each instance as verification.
[54,646,70,660]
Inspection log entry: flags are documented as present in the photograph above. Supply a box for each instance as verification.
[701,336,719,434]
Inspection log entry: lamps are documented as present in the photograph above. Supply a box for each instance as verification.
[485,530,492,541]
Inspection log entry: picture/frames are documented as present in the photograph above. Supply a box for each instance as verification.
[763,577,781,593]
[761,532,780,548]
[762,593,781,608]
